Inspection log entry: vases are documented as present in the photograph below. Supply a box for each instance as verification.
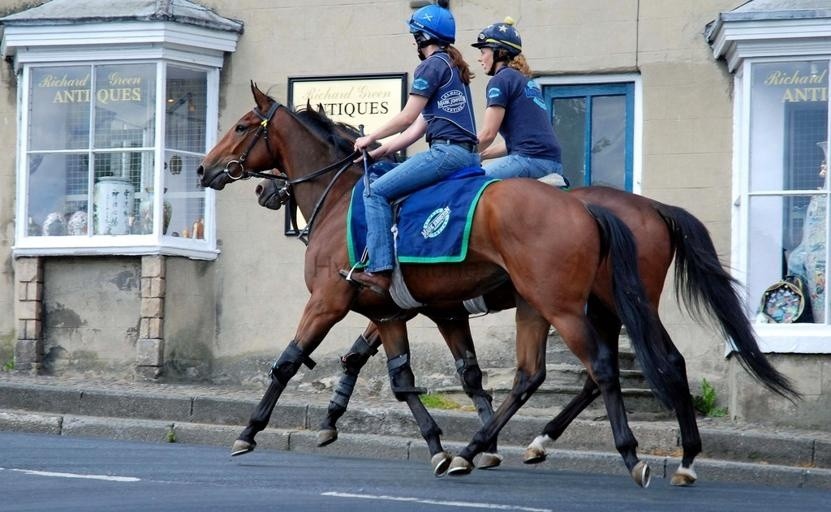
[28,176,173,236]
[802,141,828,323]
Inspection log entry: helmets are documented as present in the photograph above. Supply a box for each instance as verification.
[471,17,522,54]
[406,5,455,43]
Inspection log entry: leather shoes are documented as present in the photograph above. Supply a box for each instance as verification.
[339,269,391,297]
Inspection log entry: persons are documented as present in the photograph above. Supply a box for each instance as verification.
[337,4,480,297]
[470,16,564,177]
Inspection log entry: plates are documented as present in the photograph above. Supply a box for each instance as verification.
[758,277,806,323]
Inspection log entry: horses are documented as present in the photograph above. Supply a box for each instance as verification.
[254,94,806,489]
[192,77,687,491]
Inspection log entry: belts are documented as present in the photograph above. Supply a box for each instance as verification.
[429,139,473,151]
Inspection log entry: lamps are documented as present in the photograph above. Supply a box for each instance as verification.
[167,89,175,103]
[167,92,196,115]
